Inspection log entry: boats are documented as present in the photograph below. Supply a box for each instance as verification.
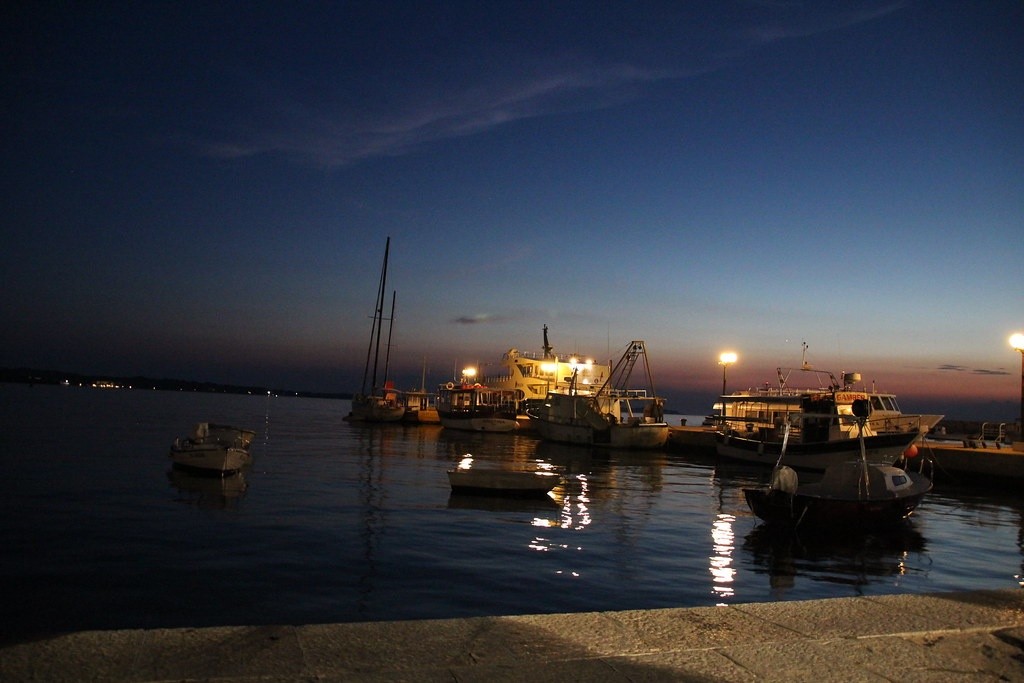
[437,381,527,433]
[404,389,440,424]
[477,347,620,423]
[168,423,255,479]
[746,402,934,528]
[704,365,946,440]
[527,341,668,450]
[713,394,920,482]
[443,465,561,498]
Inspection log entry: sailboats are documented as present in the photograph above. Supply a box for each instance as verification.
[345,237,405,422]
